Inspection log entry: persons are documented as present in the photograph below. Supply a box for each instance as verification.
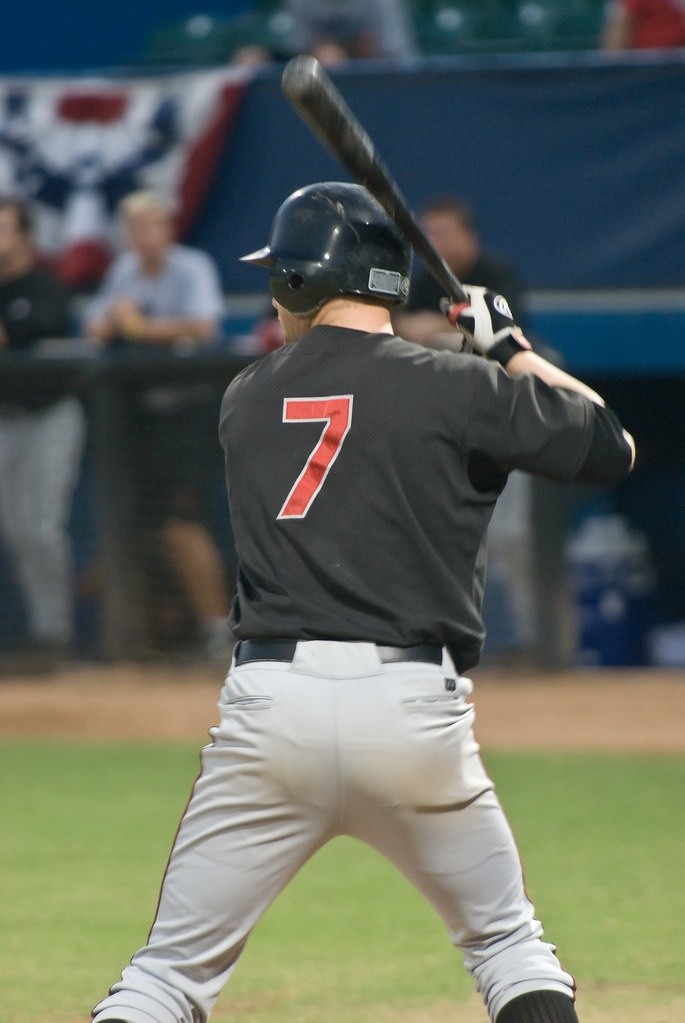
[0,194,93,677]
[416,204,585,668]
[77,190,239,668]
[83,181,637,1023]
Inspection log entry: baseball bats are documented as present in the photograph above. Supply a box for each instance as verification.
[280,54,471,305]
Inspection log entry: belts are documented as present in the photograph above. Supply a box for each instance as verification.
[234,637,443,665]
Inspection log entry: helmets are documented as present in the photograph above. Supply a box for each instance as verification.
[237,182,412,318]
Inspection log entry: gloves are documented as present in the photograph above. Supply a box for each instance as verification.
[439,284,533,369]
